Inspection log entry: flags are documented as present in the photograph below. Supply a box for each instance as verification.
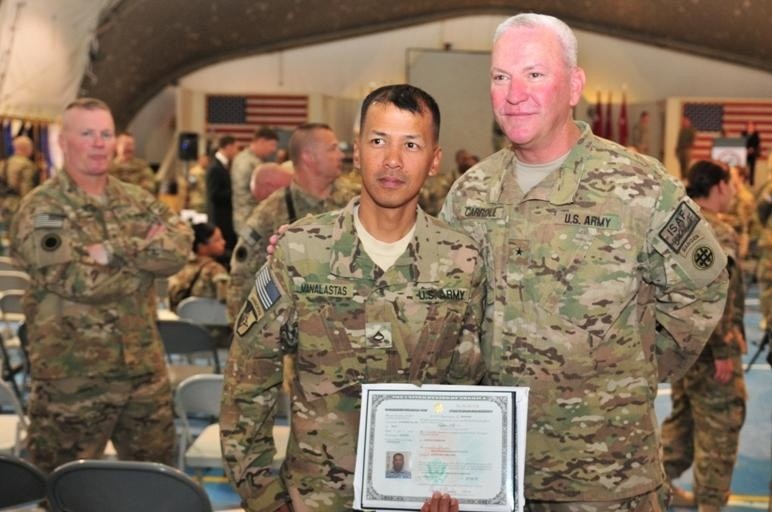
[205,95,308,157]
[618,85,629,147]
[680,101,765,176]
[594,90,602,137]
[605,91,613,142]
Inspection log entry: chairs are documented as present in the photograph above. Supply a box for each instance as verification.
[47,460,212,512]
[157,308,180,321]
[0,330,23,404]
[17,321,30,387]
[157,319,220,396]
[0,289,26,350]
[0,256,24,271]
[0,377,31,458]
[176,297,233,365]
[0,454,47,512]
[174,373,290,484]
[0,270,31,293]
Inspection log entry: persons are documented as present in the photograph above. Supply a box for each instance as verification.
[187,156,207,216]
[635,110,649,156]
[11,100,194,470]
[169,224,232,317]
[729,167,758,291]
[206,136,243,271]
[385,453,410,478]
[230,128,278,244]
[441,148,473,200]
[659,161,747,512]
[741,122,762,187]
[757,202,771,331]
[250,163,292,201]
[225,125,359,334]
[0,136,38,232]
[676,116,694,179]
[108,133,156,196]
[266,14,729,512]
[219,85,486,512]
